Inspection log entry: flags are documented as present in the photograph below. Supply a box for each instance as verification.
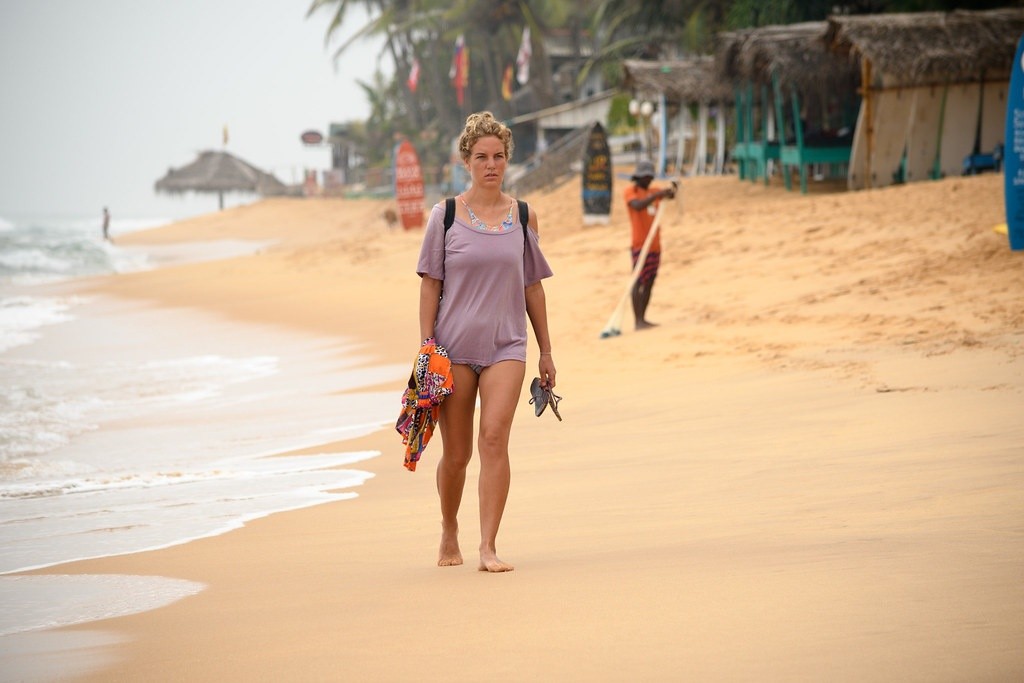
[501,60,514,100]
[449,31,470,108]
[515,22,533,85]
[405,59,422,94]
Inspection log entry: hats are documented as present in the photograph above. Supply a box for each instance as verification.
[631,162,656,181]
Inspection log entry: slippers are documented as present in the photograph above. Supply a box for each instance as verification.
[529,377,548,417]
[546,378,562,422]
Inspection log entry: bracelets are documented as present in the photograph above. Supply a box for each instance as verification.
[541,352,552,355]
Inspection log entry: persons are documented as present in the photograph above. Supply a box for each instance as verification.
[624,162,676,330]
[415,110,557,572]
[101,207,111,238]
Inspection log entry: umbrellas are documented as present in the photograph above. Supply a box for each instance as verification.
[154,150,287,213]
